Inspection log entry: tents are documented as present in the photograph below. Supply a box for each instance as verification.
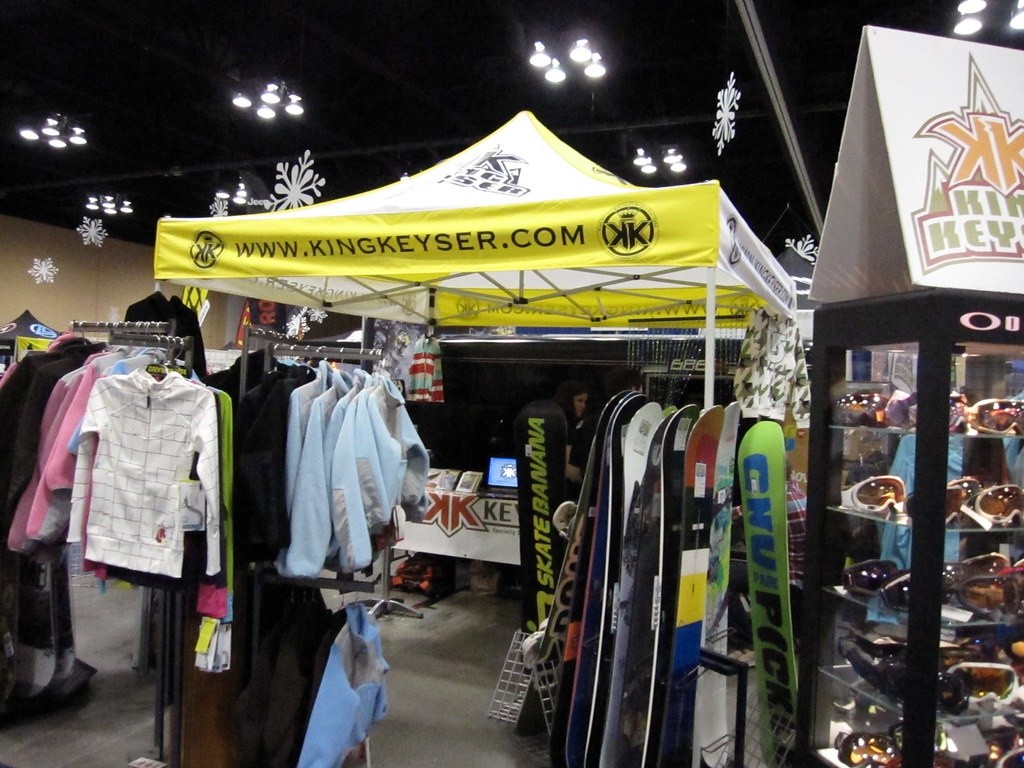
[153,109,796,412]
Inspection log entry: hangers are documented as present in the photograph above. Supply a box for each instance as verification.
[317,346,328,361]
[359,347,367,371]
[340,347,346,370]
[68,318,190,379]
[304,345,314,373]
[369,348,384,375]
[252,329,306,375]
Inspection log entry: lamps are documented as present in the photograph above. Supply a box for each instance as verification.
[523,24,609,86]
[632,141,688,179]
[229,69,306,121]
[83,187,133,216]
[217,182,250,206]
[12,103,88,151]
[950,0,1024,45]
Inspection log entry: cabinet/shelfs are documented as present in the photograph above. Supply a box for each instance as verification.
[791,286,1024,768]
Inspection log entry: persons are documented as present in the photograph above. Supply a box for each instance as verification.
[124,280,206,383]
[563,364,647,485]
[552,369,592,448]
[727,458,808,636]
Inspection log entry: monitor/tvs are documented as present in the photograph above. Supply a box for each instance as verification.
[488,457,518,488]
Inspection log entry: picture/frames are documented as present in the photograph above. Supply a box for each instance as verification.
[456,472,480,493]
[467,470,485,492]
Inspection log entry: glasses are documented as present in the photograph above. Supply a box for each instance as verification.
[831,391,1024,768]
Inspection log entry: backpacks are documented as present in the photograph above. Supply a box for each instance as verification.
[391,552,455,597]
